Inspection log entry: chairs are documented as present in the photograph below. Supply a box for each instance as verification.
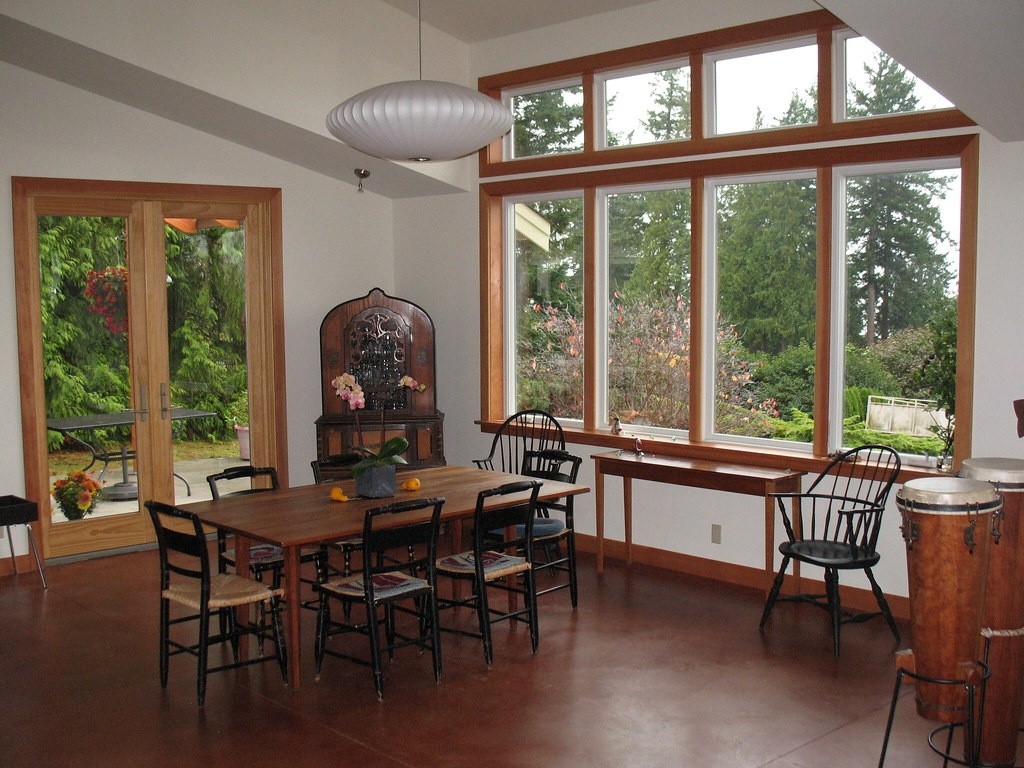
[0,495,46,588]
[759,443,902,657]
[145,408,584,706]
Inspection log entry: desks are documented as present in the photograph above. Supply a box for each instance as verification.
[590,451,809,604]
[177,464,589,690]
[47,407,217,500]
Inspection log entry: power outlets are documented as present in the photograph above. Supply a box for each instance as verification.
[712,524,721,544]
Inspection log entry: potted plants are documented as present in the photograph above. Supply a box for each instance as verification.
[223,390,250,460]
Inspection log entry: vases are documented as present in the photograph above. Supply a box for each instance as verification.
[353,464,396,498]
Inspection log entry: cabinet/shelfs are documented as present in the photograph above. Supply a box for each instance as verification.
[311,289,447,485]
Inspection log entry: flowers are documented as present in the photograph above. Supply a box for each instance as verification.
[332,373,425,476]
[87,268,129,333]
[50,470,107,520]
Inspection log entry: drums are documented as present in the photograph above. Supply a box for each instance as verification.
[958,455,1024,768]
[897,476,1004,725]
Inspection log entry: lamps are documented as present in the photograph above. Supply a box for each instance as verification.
[325,0,515,162]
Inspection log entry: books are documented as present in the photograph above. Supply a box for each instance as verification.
[440,550,526,572]
[337,574,426,596]
